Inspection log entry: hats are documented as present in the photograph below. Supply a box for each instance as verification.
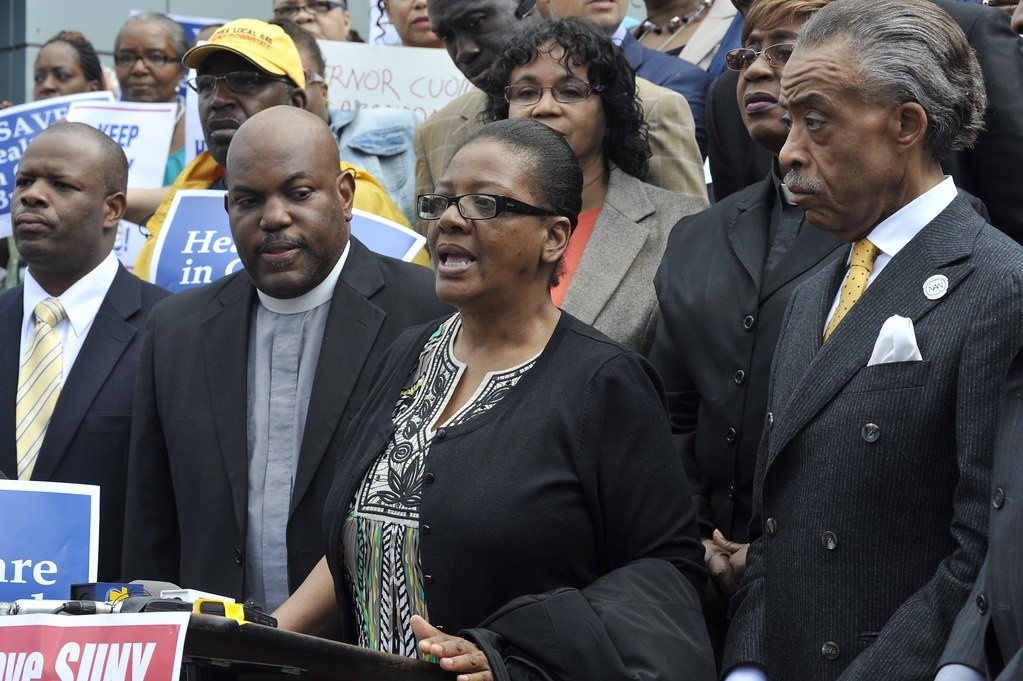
[181,18,306,91]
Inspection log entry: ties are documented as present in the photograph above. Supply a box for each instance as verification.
[823,238,879,353]
[15,297,68,478]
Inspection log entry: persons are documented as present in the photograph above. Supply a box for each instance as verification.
[0,0,1023,680]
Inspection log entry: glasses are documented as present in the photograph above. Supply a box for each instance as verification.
[115,51,178,67]
[416,193,551,221]
[503,80,592,106]
[725,43,795,72]
[187,70,294,97]
[274,0,342,18]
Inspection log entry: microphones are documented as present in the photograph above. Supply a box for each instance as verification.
[63,601,122,615]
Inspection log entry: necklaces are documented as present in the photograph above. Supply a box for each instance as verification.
[637,5,705,52]
[643,0,713,36]
